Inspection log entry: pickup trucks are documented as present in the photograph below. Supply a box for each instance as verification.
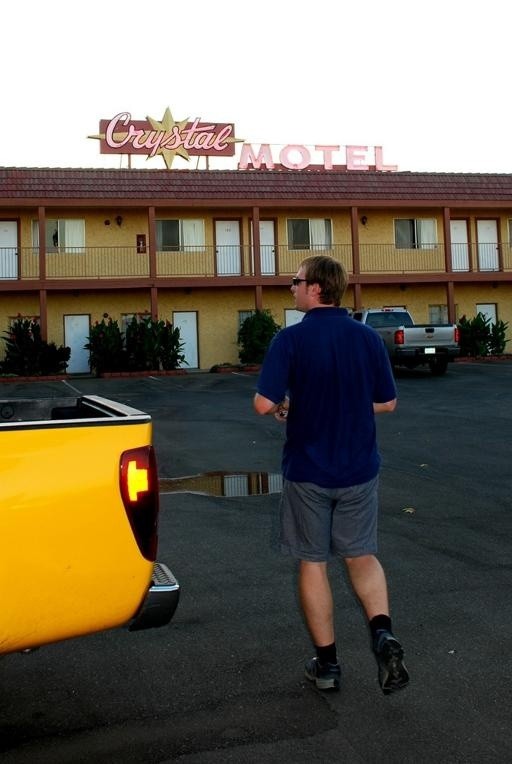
[347,306,463,376]
[0,393,181,655]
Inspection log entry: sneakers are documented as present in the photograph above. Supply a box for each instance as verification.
[304,657,342,692]
[374,633,410,696]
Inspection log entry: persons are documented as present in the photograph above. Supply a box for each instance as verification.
[254,254,409,695]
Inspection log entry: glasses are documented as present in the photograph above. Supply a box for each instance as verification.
[292,277,306,285]
